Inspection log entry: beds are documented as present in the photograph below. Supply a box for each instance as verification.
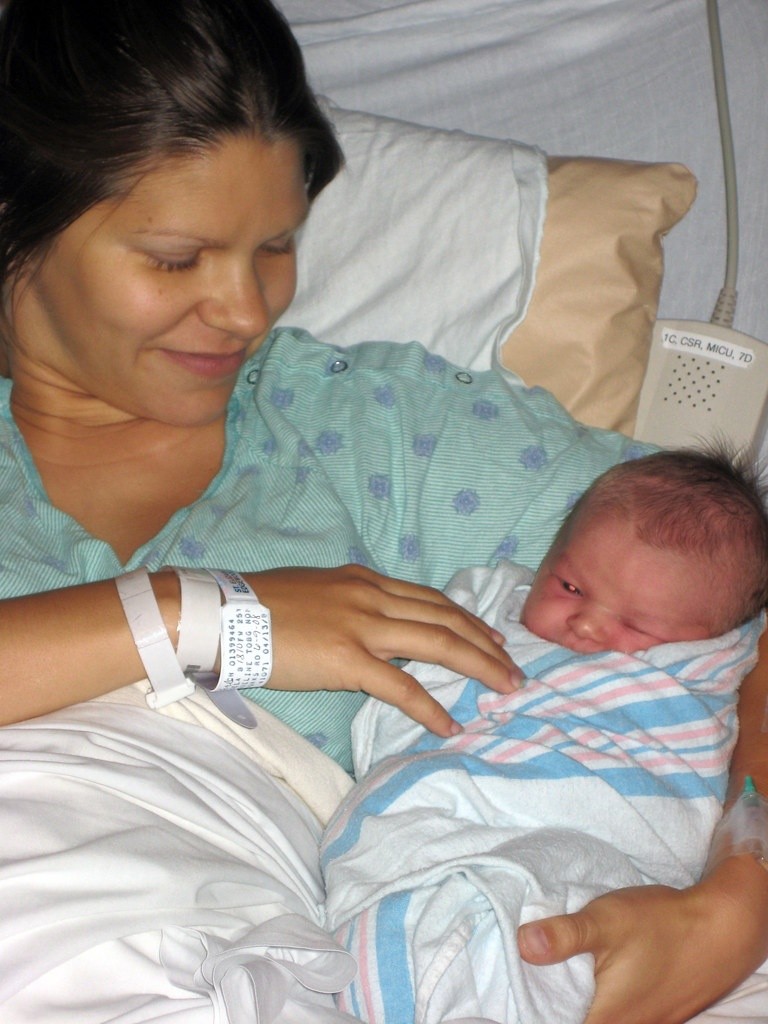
[0,0,768,1024]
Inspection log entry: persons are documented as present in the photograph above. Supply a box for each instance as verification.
[0,0,768,1024]
[306,448,768,1024]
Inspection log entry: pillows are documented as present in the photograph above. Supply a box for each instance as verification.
[271,94,697,439]
